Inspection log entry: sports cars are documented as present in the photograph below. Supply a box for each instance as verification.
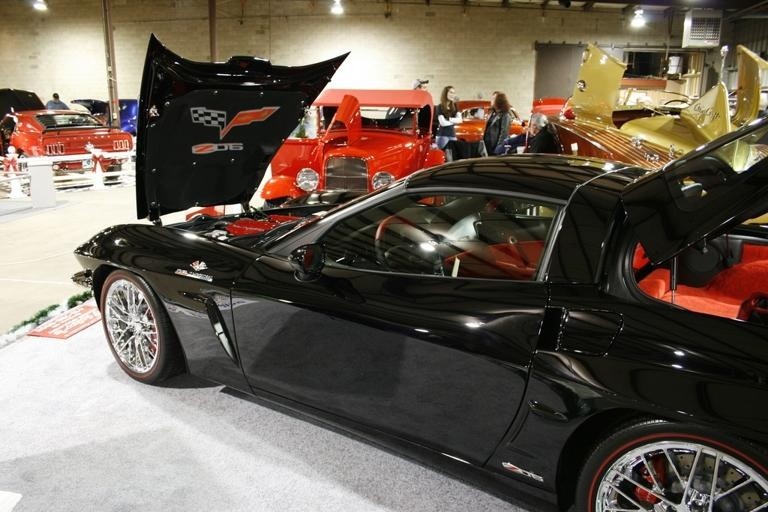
[0,88,140,181]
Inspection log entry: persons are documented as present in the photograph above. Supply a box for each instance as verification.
[45,92,71,110]
[386,78,562,161]
